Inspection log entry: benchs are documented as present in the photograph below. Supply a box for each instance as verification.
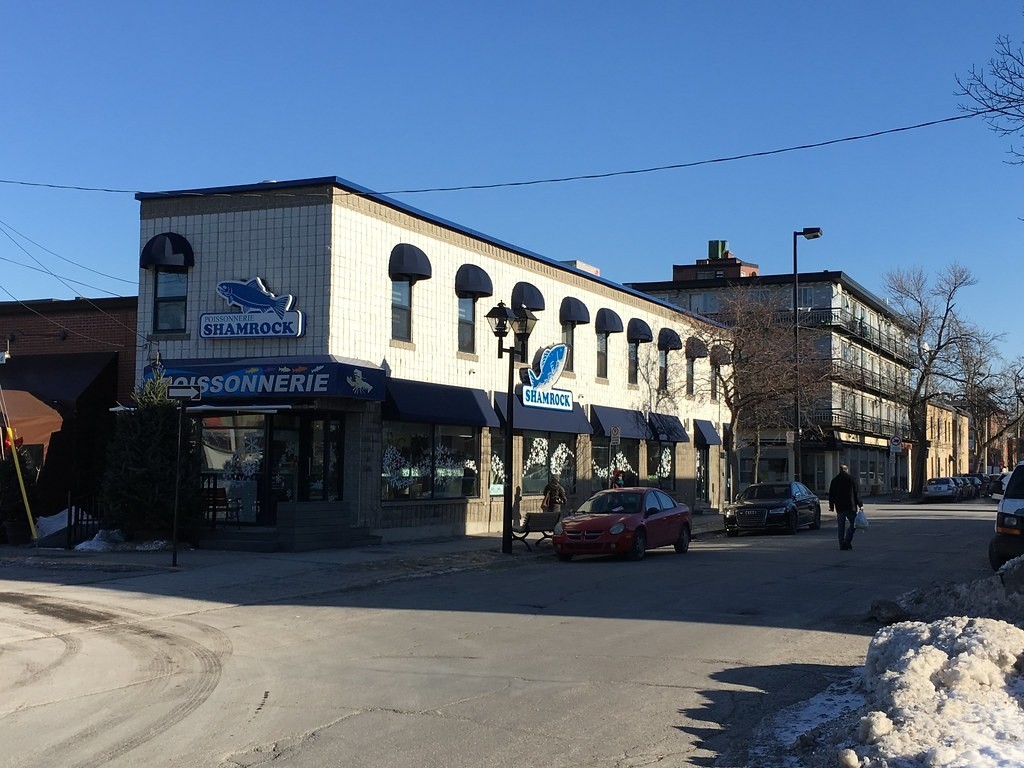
[201,487,243,529]
[511,512,570,553]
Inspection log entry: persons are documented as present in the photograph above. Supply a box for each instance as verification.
[543,474,567,512]
[610,468,623,489]
[828,465,863,550]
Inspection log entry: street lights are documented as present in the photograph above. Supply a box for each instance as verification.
[792,226,823,483]
[484,298,539,555]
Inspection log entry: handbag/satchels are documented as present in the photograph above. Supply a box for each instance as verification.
[853,509,870,530]
[552,496,564,505]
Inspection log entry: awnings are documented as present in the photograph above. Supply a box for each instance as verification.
[658,329,682,351]
[382,377,501,428]
[595,308,623,333]
[493,392,593,435]
[389,244,432,278]
[710,345,731,366]
[723,423,744,448]
[456,264,492,296]
[591,404,654,440]
[693,420,722,445]
[513,282,545,312]
[628,318,653,343]
[685,337,708,358]
[560,297,590,325]
[0,352,118,410]
[649,412,690,442]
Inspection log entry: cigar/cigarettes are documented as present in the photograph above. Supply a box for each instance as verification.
[829,510,832,511]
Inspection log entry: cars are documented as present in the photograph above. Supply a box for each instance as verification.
[922,470,1013,503]
[723,480,822,538]
[552,486,692,562]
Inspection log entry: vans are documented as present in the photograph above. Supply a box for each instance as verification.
[987,460,1024,573]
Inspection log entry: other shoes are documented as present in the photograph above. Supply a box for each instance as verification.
[843,541,852,549]
[840,546,845,550]
